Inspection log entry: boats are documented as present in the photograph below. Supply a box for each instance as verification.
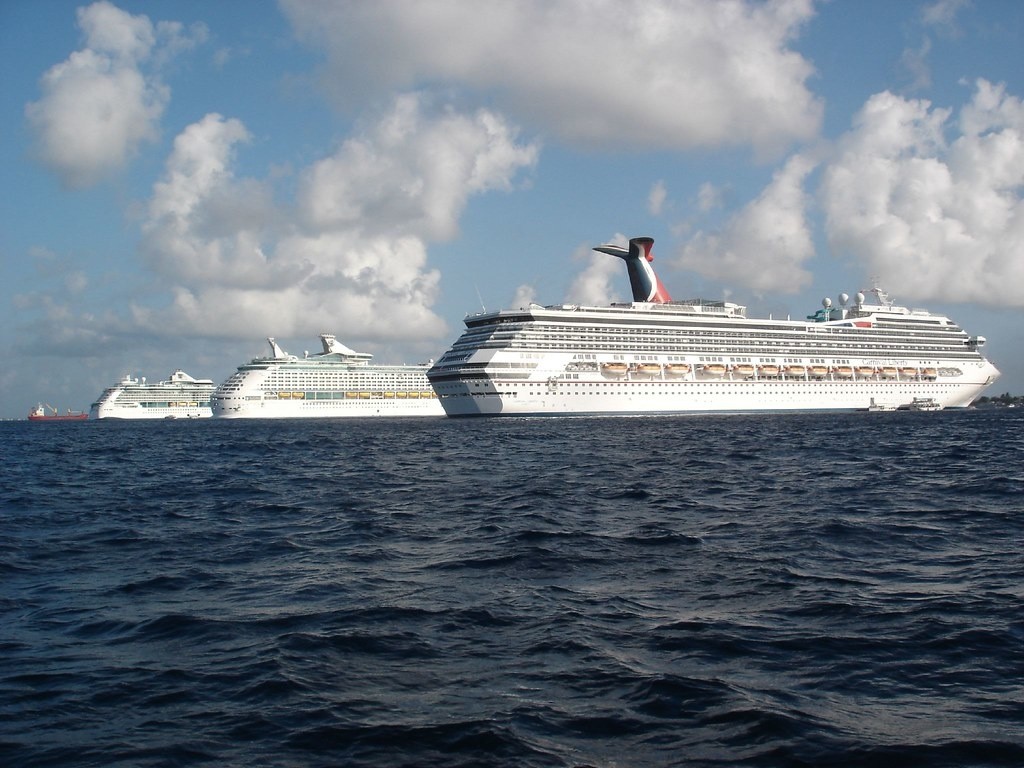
[900,369,919,378]
[384,393,394,397]
[636,364,661,375]
[785,367,804,376]
[279,392,291,397]
[420,393,430,397]
[408,393,418,398]
[868,405,896,411]
[909,397,945,412]
[879,369,897,378]
[168,402,198,407]
[922,370,937,379]
[359,392,369,398]
[664,364,690,375]
[346,392,357,398]
[855,368,874,378]
[833,369,852,377]
[703,365,725,375]
[733,366,753,375]
[27,402,89,420]
[601,364,626,375]
[807,369,828,377]
[758,367,778,376]
[396,392,406,397]
[293,392,304,398]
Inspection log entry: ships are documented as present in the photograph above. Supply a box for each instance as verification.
[209,333,448,418]
[425,236,1002,413]
[87,369,219,422]
